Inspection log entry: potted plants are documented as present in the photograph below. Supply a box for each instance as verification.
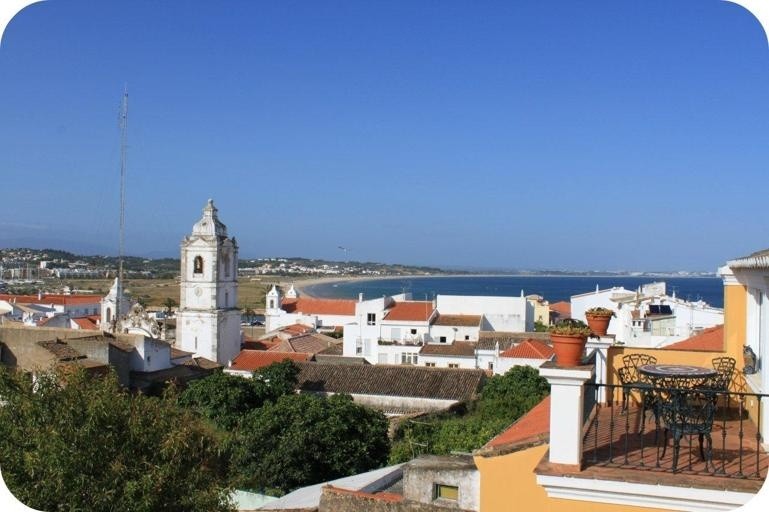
[549,307,617,369]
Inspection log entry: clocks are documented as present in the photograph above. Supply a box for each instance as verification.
[194,287,203,297]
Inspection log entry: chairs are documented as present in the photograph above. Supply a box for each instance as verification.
[615,352,750,472]
